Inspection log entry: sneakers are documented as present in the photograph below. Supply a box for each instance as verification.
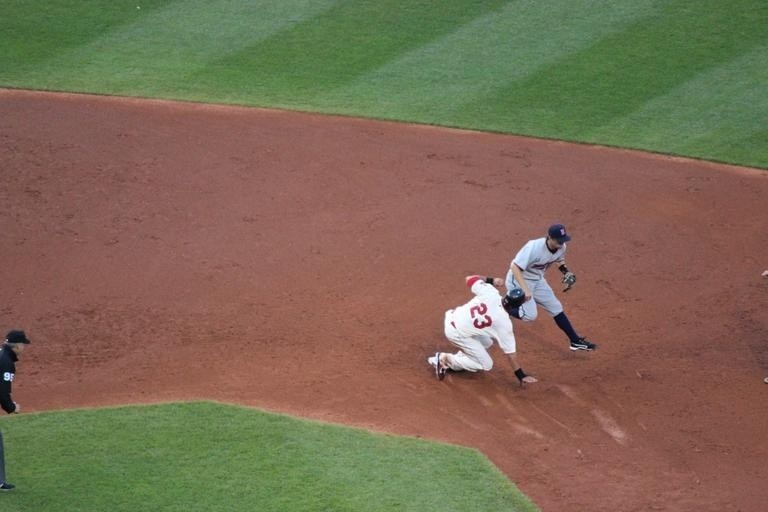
[427,357,435,367]
[435,351,450,381]
[569,334,600,352]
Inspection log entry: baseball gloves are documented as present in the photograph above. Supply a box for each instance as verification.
[561,271,575,293]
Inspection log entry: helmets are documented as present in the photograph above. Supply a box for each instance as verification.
[506,288,525,309]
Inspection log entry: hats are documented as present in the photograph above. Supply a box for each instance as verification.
[547,223,572,244]
[0,481,16,491]
[4,329,32,345]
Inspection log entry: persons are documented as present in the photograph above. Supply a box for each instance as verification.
[0,330,29,491]
[426,273,538,383]
[504,223,597,351]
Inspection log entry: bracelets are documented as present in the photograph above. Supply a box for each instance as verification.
[514,368,527,380]
[485,277,494,284]
